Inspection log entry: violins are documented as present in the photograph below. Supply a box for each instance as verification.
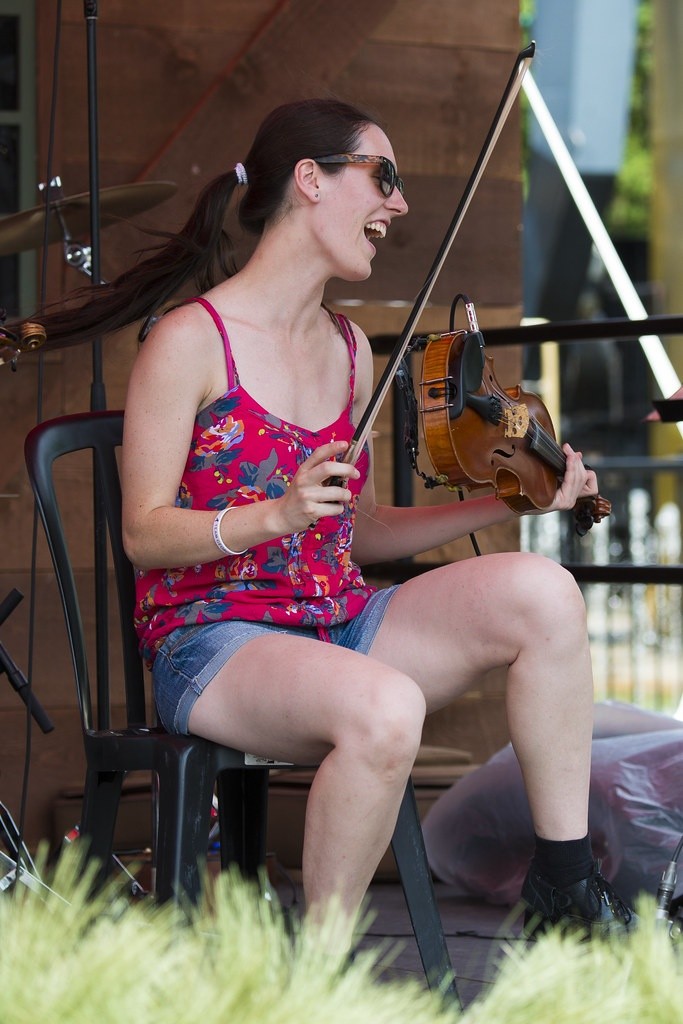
[417,329,611,538]
[0,308,47,373]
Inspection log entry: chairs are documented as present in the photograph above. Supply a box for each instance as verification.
[23,409,464,1023]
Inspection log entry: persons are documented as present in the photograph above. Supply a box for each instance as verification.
[0,88,637,970]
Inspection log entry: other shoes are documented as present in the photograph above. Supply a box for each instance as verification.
[518,859,646,942]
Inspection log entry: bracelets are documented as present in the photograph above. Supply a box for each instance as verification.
[213,505,249,555]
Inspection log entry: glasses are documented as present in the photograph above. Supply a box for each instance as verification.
[314,154,407,198]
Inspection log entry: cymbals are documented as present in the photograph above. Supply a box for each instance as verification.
[0,181,179,258]
